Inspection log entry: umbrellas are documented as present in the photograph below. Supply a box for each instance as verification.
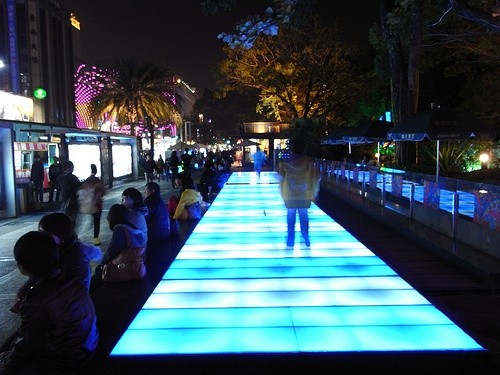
[321,106,496,184]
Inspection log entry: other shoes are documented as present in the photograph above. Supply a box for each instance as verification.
[303,244,310,250]
[285,243,294,250]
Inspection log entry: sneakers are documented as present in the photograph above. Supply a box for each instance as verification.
[93,237,101,245]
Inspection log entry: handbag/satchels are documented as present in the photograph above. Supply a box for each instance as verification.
[101,225,135,283]
[187,201,202,220]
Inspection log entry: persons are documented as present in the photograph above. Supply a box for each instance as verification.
[249,147,267,179]
[79,164,105,246]
[138,145,240,193]
[89,203,147,292]
[48,156,63,201]
[56,160,82,231]
[275,127,322,250]
[121,188,150,242]
[0,231,100,375]
[38,213,105,294]
[144,182,171,243]
[31,155,44,202]
[166,170,211,223]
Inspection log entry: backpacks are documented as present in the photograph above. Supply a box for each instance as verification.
[75,185,99,214]
